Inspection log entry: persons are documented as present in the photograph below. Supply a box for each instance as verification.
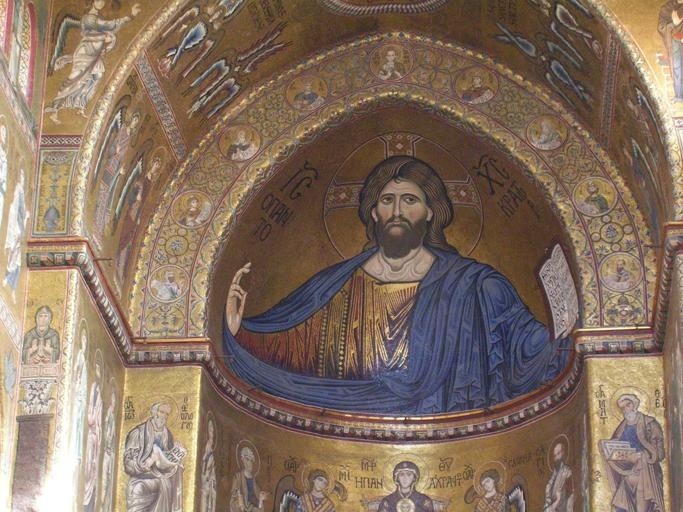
[459,71,496,105]
[288,78,324,111]
[531,120,563,150]
[176,196,203,227]
[1,166,32,305]
[598,386,667,512]
[153,268,180,301]
[224,131,260,160]
[374,459,434,511]
[224,439,271,511]
[376,48,406,83]
[109,154,164,297]
[21,307,59,365]
[70,318,189,510]
[40,1,141,127]
[657,0,683,101]
[578,180,610,214]
[604,259,638,291]
[92,105,142,252]
[0,114,8,220]
[199,409,218,511]
[472,459,511,511]
[221,155,577,422]
[278,464,340,512]
[619,84,674,245]
[541,435,576,510]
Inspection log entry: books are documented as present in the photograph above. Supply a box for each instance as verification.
[598,436,643,477]
